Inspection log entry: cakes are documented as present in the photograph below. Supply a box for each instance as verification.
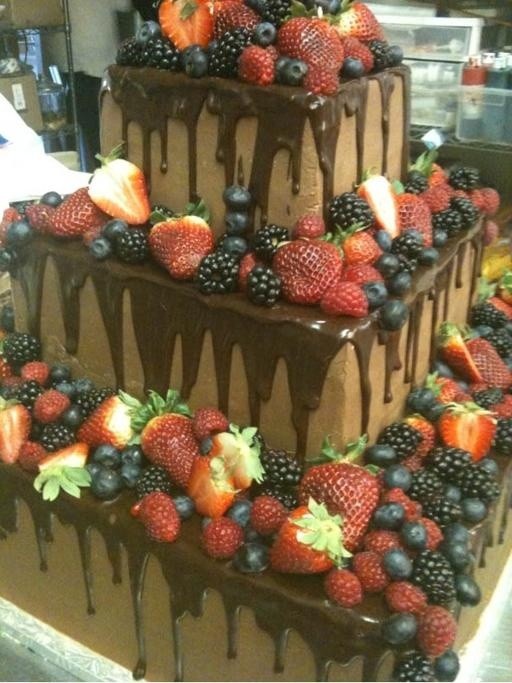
[0,0,510,680]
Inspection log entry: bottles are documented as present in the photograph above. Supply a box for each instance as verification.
[457,50,512,144]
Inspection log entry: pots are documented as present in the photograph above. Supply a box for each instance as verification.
[36,76,65,114]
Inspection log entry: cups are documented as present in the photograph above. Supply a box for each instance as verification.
[1,29,18,60]
